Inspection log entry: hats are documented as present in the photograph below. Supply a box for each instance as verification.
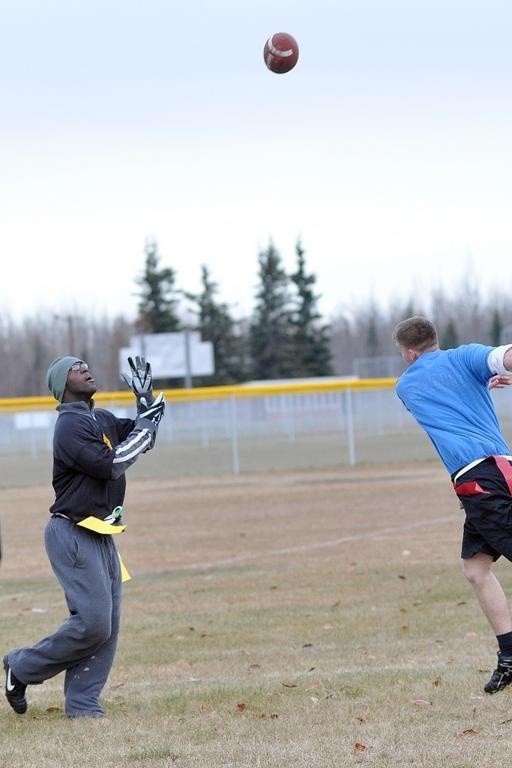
[46,356,84,402]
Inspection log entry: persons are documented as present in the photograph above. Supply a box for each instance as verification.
[387,313,512,696]
[1,352,167,724]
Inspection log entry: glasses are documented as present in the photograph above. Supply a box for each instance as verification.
[72,363,88,371]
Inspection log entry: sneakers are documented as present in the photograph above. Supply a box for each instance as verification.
[484,651,512,693]
[2,654,28,713]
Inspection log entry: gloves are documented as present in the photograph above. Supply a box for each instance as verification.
[121,356,153,399]
[138,393,166,428]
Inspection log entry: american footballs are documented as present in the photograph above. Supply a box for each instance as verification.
[264,34,298,72]
